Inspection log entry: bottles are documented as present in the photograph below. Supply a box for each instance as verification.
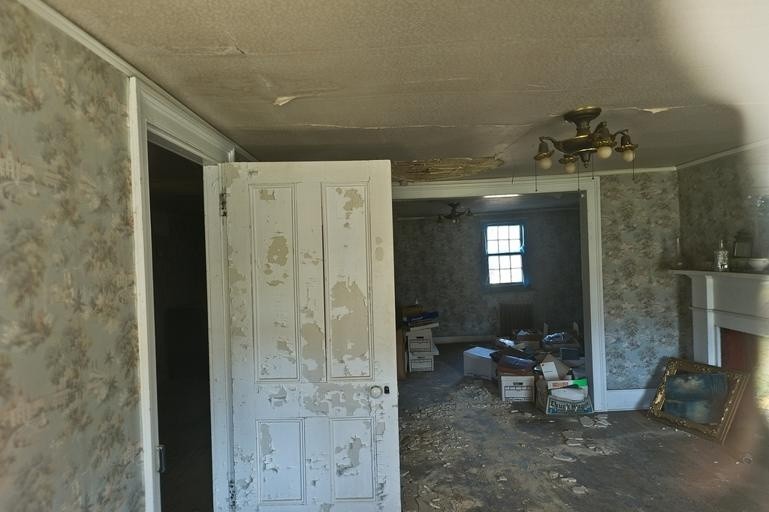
[564,367,574,380]
[733,228,752,255]
[671,236,687,268]
[712,238,729,272]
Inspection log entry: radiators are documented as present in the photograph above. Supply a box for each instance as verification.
[496,302,536,336]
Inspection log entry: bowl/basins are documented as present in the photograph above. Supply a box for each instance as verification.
[730,257,768,272]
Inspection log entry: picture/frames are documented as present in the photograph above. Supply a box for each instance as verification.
[645,356,752,448]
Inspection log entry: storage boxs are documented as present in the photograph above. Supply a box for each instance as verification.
[463,322,595,416]
[401,303,440,373]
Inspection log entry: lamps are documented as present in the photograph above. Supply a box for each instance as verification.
[533,106,640,174]
[436,201,474,226]
